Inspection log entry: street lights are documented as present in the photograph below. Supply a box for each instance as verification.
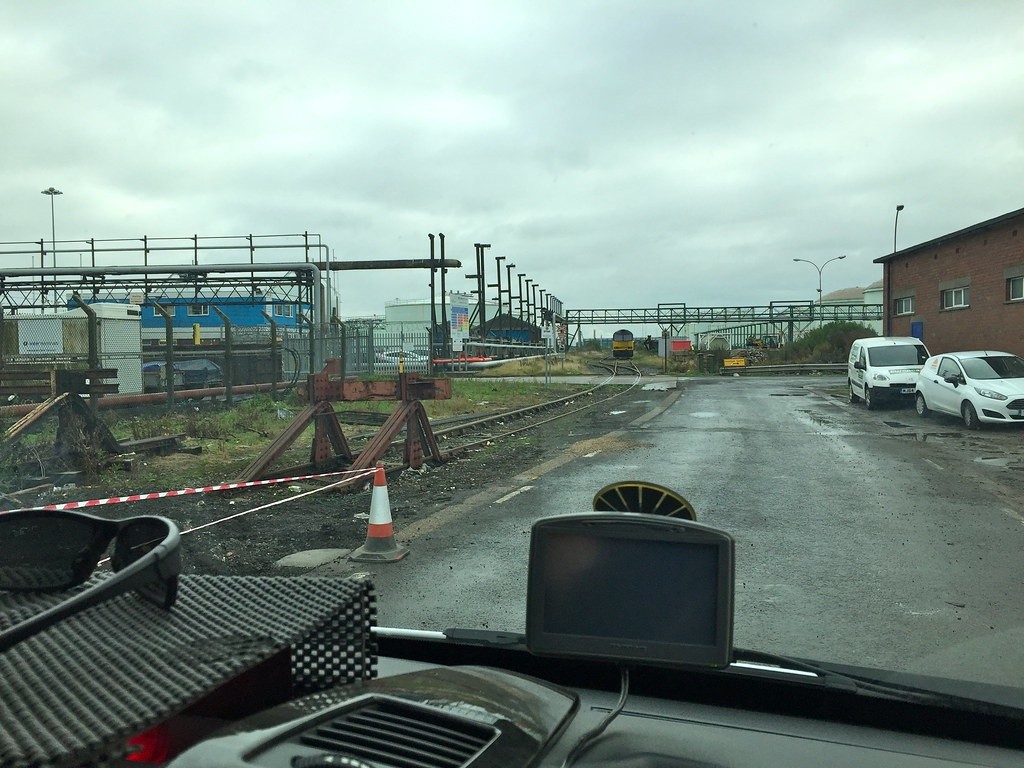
[793,255,847,328]
[894,204,905,252]
[40,186,65,313]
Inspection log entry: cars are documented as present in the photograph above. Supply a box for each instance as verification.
[914,350,1024,429]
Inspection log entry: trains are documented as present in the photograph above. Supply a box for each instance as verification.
[612,329,635,359]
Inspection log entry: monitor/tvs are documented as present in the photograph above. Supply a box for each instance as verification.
[525,511,736,668]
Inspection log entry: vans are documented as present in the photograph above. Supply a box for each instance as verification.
[847,336,932,411]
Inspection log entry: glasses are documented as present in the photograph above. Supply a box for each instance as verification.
[0,510,183,653]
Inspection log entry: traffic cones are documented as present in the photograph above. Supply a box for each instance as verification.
[349,460,411,565]
